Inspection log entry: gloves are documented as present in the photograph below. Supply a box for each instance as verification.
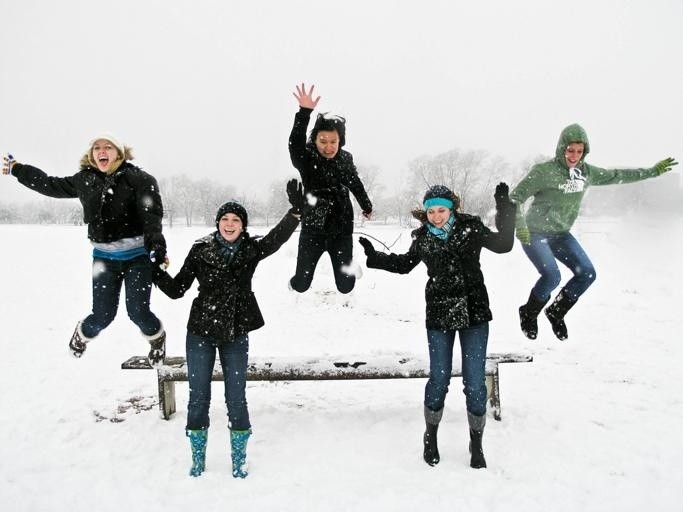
[160,254,169,269]
[495,183,509,209]
[359,237,375,257]
[655,158,677,174]
[1,153,16,175]
[516,227,529,245]
[286,179,304,215]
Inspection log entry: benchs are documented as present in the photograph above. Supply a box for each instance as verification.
[121,353,532,421]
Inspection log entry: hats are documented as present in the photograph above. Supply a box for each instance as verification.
[423,185,457,209]
[88,135,125,154]
[215,202,248,234]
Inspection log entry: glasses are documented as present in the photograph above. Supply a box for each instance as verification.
[318,113,346,123]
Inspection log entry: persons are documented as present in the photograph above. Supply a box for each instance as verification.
[1,131,171,362]
[145,179,307,479]
[359,182,519,470]
[287,81,373,293]
[509,123,678,342]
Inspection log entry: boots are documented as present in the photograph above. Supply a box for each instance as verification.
[70,321,90,352]
[144,319,166,368]
[230,431,252,478]
[545,288,578,340]
[467,410,486,468]
[519,289,550,339]
[424,404,443,466]
[185,430,208,477]
